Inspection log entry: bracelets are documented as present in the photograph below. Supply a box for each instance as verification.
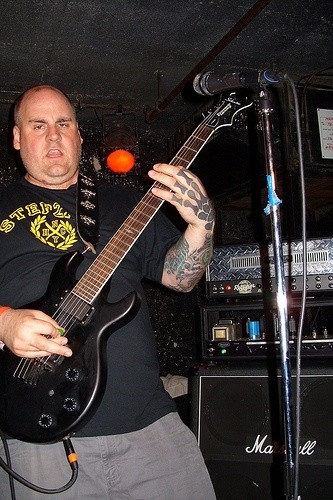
[0,306,10,315]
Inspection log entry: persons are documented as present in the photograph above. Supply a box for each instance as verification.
[0,84,216,500]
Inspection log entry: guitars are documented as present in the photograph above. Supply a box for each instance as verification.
[0,95,254,445]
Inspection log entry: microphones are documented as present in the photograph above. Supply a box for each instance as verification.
[193,68,282,96]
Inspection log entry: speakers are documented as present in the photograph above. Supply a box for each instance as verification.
[190,366,333,500]
